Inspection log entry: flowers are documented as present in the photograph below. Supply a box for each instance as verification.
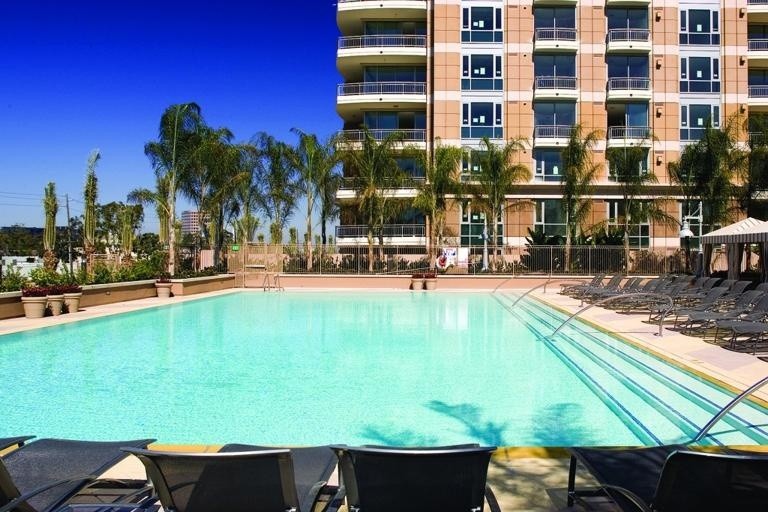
[412,271,436,278]
[155,276,171,282]
[20,284,82,297]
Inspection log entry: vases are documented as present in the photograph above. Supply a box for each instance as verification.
[412,278,437,290]
[21,293,82,318]
[155,282,173,298]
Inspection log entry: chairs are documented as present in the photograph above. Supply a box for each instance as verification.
[0,437,38,452]
[559,273,768,355]
[326,442,502,511]
[565,443,768,511]
[0,439,158,511]
[120,443,348,511]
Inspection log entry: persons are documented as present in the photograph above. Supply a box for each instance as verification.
[105,244,198,274]
[283,253,429,274]
[468,251,530,275]
